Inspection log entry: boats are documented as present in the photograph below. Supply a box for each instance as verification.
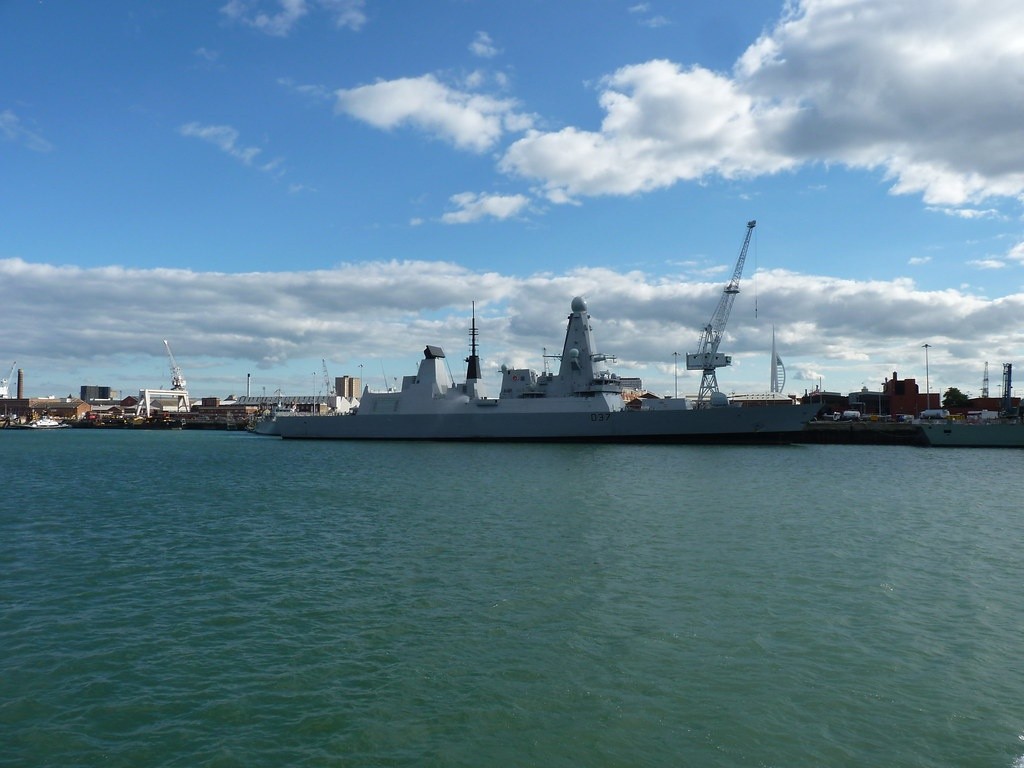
[68,413,176,430]
[13,415,69,429]
[181,418,251,430]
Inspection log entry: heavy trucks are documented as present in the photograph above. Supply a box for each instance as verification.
[919,409,951,419]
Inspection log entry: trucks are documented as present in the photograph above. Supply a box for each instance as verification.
[967,409,1001,421]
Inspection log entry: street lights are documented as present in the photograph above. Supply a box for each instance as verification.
[922,343,932,410]
[358,364,364,396]
[671,351,681,400]
[312,372,317,416]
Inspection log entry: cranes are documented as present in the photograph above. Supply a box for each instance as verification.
[0,361,17,399]
[685,219,759,410]
[163,339,186,391]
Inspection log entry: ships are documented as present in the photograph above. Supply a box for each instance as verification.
[254,294,827,446]
